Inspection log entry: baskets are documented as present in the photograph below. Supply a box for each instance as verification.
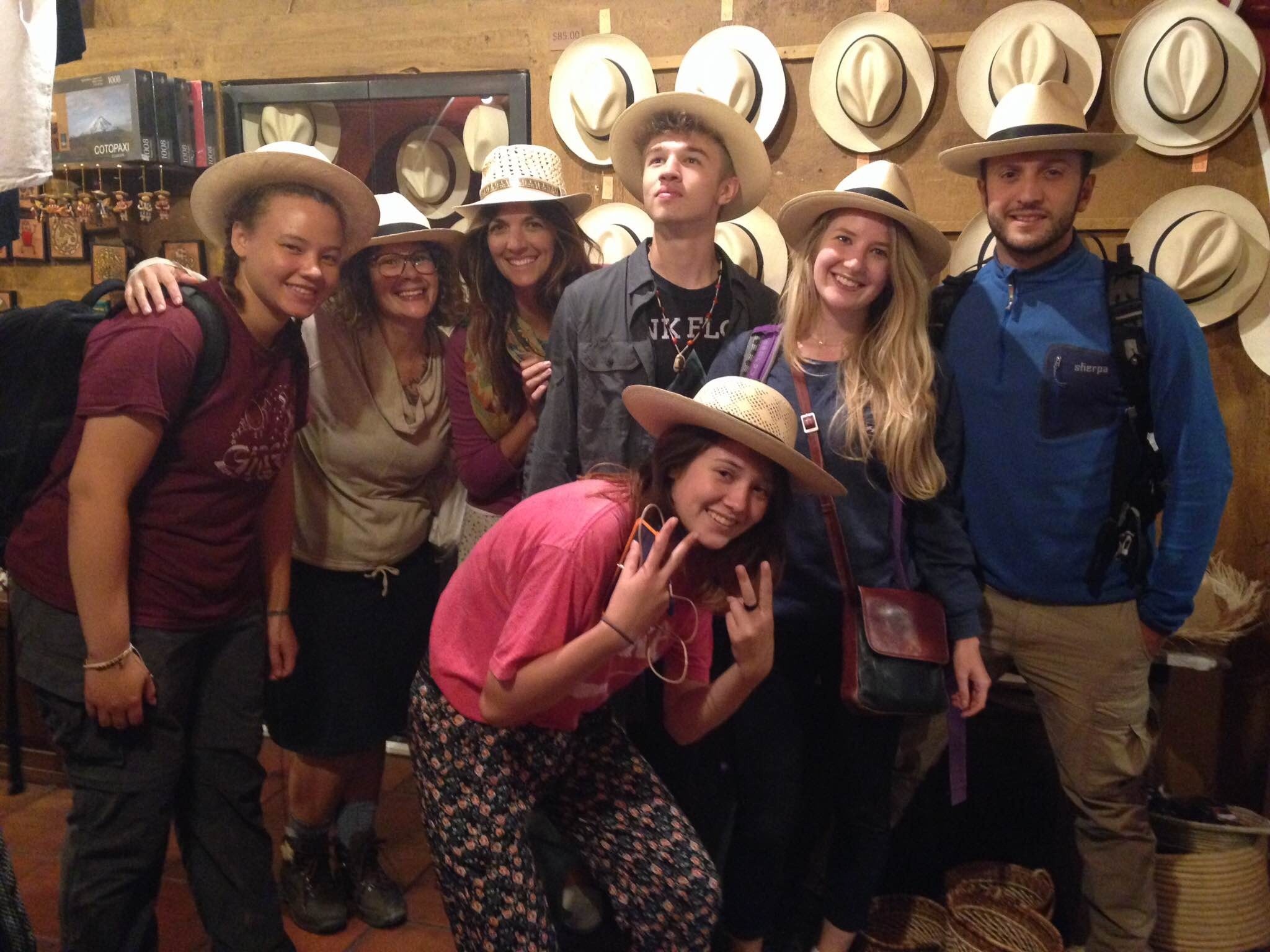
[858,864,1062,952]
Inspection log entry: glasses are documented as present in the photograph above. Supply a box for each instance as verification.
[365,250,440,276]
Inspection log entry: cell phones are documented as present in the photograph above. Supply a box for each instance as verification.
[605,518,658,598]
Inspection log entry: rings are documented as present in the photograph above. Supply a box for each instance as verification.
[743,601,758,611]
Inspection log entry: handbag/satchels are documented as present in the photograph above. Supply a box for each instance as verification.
[841,584,953,717]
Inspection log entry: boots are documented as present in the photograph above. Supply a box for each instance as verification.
[278,832,347,934]
[333,833,407,928]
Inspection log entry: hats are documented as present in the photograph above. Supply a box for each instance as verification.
[776,159,952,278]
[674,24,787,142]
[955,0,1103,138]
[452,144,592,224]
[1237,265,1270,376]
[937,80,1139,176]
[1110,0,1265,156]
[190,141,381,262]
[394,125,471,219]
[240,101,342,163]
[549,34,658,166]
[577,202,655,264]
[609,92,771,223]
[1123,185,1270,328]
[948,207,997,276]
[714,205,788,294]
[623,374,848,496]
[372,191,476,252]
[809,11,934,153]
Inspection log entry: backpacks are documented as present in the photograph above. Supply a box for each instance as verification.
[0,278,311,544]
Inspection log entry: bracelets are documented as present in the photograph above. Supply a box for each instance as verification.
[84,643,132,670]
[601,615,636,645]
[266,609,291,616]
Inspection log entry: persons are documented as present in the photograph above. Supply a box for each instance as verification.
[4,144,377,952]
[925,79,1233,952]
[123,192,459,928]
[406,94,992,952]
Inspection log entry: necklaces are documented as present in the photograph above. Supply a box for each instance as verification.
[805,332,841,348]
[648,243,722,372]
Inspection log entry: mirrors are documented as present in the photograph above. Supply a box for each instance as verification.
[216,68,534,231]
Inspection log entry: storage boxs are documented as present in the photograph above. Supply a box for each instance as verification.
[48,66,220,169]
